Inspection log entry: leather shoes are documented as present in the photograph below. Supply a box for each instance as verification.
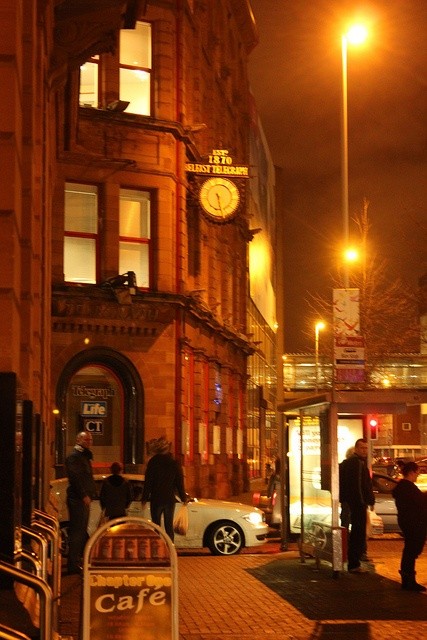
[68,567,83,572]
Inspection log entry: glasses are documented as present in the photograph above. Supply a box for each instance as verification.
[413,472,420,475]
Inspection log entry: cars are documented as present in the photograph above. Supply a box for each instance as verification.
[44,474,270,556]
[274,470,404,553]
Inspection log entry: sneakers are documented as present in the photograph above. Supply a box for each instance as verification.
[360,554,372,562]
[349,566,370,573]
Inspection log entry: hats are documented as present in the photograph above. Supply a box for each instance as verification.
[152,437,171,453]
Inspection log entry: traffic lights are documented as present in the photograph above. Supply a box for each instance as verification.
[369,418,377,440]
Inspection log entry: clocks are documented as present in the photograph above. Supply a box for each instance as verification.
[198,177,241,220]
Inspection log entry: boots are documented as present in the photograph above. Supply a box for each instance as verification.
[399,570,425,591]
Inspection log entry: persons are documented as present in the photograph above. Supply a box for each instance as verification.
[100,462,132,526]
[66,432,96,572]
[340,439,375,573]
[143,439,187,543]
[391,462,427,590]
[342,448,369,561]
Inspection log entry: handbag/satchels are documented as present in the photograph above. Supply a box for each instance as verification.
[98,511,106,528]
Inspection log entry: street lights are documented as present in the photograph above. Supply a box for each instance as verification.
[335,7,378,291]
[313,321,326,395]
[342,243,364,290]
